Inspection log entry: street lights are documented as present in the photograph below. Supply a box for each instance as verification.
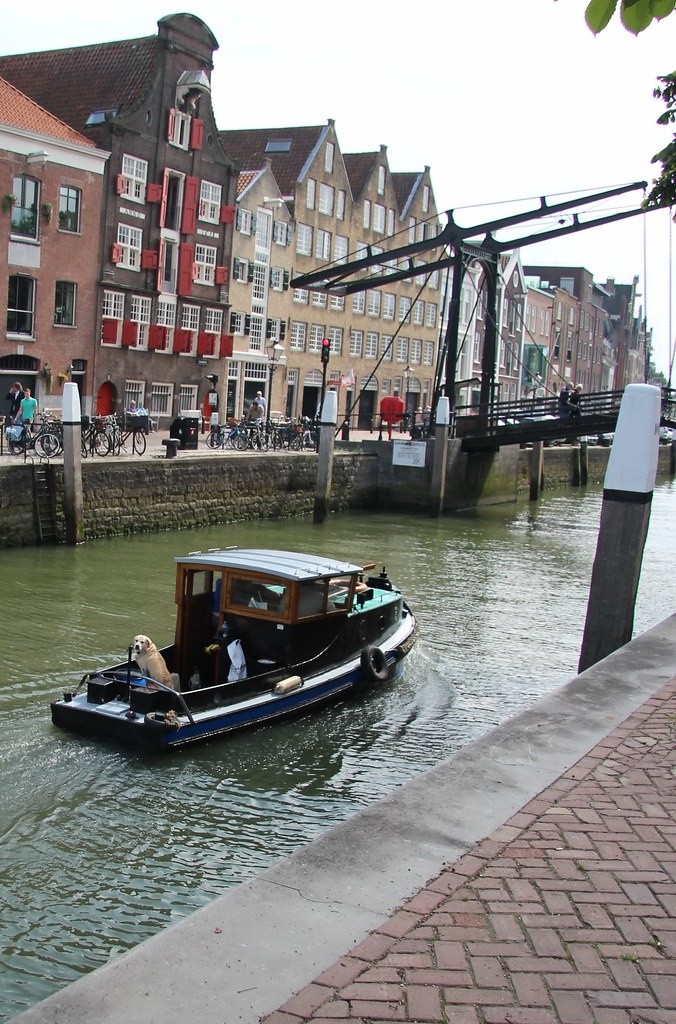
[531,372,543,416]
[265,340,285,451]
[403,365,414,433]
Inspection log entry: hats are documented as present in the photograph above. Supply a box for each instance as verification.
[255,391,262,396]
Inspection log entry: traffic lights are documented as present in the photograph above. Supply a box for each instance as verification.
[206,374,218,383]
[322,339,332,363]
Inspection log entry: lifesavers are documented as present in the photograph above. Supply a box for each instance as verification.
[329,580,368,594]
[361,647,388,679]
[144,711,180,733]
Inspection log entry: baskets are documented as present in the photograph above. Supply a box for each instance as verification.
[95,417,106,431]
[81,417,89,430]
[127,415,148,431]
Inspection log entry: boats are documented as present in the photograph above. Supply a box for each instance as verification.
[52,548,422,756]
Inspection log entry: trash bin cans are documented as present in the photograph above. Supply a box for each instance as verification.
[169,415,199,450]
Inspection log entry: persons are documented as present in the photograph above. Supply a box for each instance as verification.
[5,382,37,434]
[254,391,267,416]
[415,406,432,423]
[246,401,264,452]
[127,399,155,432]
[558,382,583,417]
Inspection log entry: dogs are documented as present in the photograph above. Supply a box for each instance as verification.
[133,635,175,690]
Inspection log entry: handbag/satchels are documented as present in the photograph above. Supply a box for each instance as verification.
[228,428,237,439]
[6,425,24,441]
[227,639,248,683]
[248,597,267,610]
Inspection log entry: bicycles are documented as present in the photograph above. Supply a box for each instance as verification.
[206,416,318,451]
[7,411,148,458]
